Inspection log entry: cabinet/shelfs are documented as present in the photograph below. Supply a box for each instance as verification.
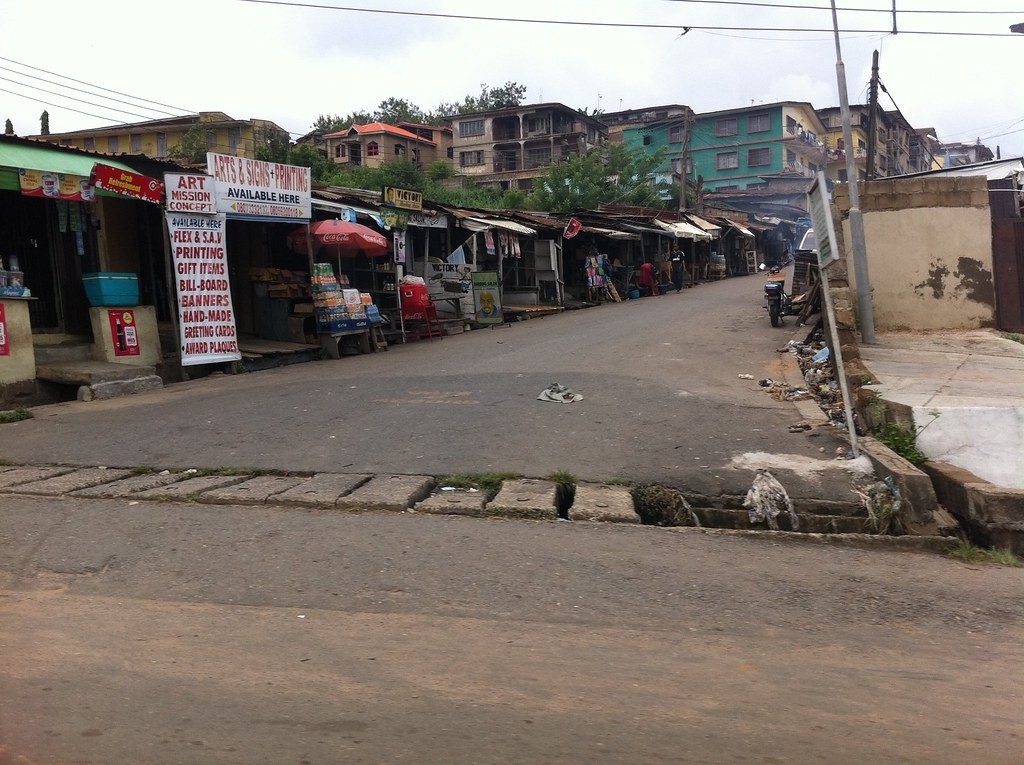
[400,299,442,343]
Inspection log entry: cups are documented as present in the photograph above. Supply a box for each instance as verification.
[80,180,90,197]
[42,175,57,194]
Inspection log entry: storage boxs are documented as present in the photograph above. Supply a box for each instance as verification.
[400,283,430,307]
[245,261,383,334]
[79,270,142,309]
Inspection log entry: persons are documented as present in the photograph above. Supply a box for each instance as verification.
[665,242,687,293]
[638,263,660,296]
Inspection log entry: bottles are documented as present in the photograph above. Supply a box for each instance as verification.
[116,318,126,353]
[312,262,395,330]
[0,255,24,287]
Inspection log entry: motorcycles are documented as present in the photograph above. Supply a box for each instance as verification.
[758,259,793,328]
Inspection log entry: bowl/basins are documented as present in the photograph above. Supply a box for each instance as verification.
[629,289,639,299]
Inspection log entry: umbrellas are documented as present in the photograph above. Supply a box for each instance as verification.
[288,219,393,275]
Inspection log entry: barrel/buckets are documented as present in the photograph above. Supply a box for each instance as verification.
[399,283,430,306]
